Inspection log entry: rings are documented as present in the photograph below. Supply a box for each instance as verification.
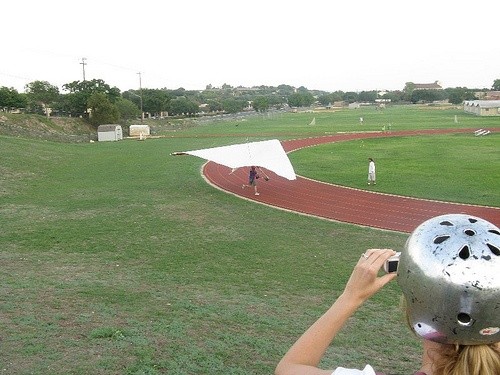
[361,253,368,260]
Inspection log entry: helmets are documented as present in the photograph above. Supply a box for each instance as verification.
[397,214,500,345]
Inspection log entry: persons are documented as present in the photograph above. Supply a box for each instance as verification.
[367,158,376,185]
[242,166,260,196]
[277,213,500,375]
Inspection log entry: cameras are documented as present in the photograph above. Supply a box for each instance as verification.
[383,252,401,274]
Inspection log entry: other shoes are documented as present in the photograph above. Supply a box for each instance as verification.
[255,193,259,196]
[242,184,245,189]
